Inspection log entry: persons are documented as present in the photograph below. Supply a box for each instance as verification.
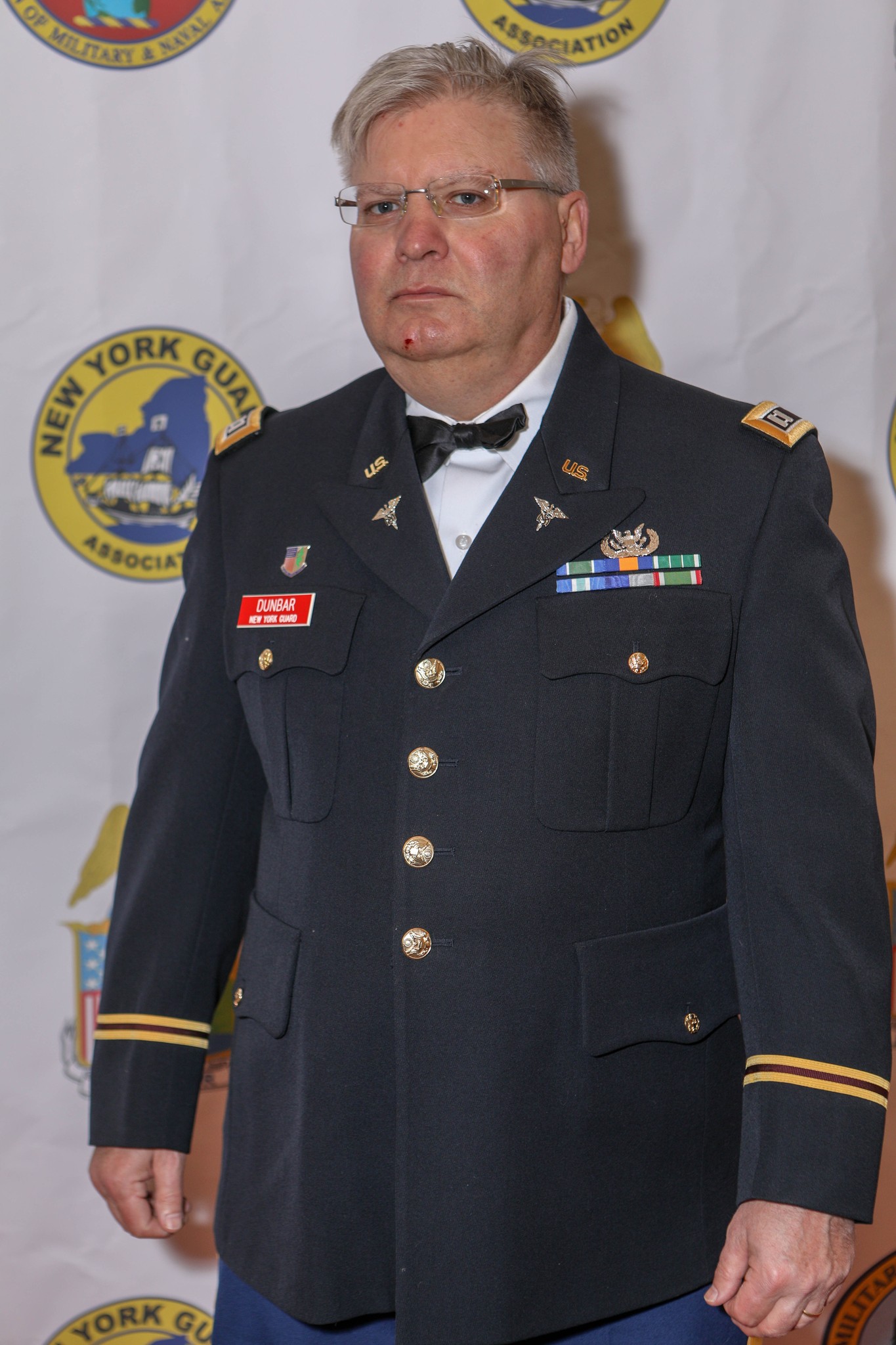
[87,38,895,1345]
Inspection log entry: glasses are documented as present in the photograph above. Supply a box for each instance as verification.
[334,174,564,226]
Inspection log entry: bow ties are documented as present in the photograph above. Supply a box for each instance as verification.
[406,403,526,483]
